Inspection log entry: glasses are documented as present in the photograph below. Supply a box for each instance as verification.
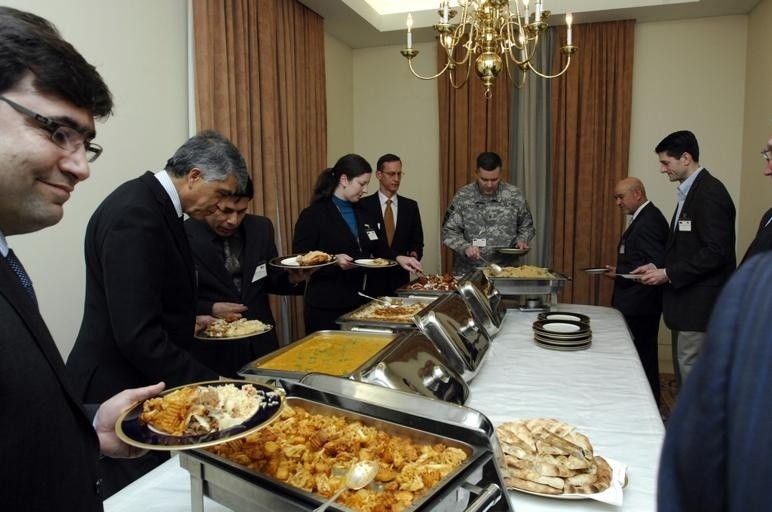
[381,171,404,176]
[760,150,771,160]
[0,96,103,163]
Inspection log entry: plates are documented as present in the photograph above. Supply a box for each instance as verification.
[614,273,644,279]
[532,311,592,351]
[581,267,610,274]
[268,254,338,269]
[349,257,397,269]
[194,324,274,340]
[114,380,283,453]
[490,246,531,254]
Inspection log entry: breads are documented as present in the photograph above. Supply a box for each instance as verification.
[492,415,613,494]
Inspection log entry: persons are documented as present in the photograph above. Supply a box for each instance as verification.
[179,170,322,378]
[353,154,425,295]
[62,128,252,500]
[655,251,772,510]
[603,177,670,408]
[293,154,429,333]
[627,131,736,401]
[1,6,169,511]
[440,152,537,277]
[732,137,772,267]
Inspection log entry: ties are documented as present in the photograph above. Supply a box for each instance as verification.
[6,248,38,312]
[383,200,395,248]
[224,239,241,273]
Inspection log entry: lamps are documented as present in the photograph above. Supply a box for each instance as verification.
[403,0,579,101]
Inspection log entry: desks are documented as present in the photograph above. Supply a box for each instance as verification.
[101,303,671,510]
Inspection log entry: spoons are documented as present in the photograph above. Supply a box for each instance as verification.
[311,459,380,511]
